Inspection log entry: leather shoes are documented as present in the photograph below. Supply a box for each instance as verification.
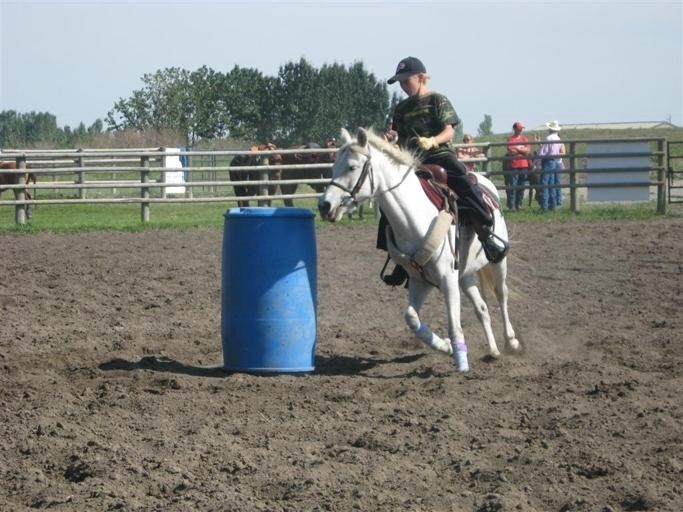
[481,234,505,262]
[383,265,405,284]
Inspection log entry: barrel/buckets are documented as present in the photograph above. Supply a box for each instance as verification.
[220,207,320,375]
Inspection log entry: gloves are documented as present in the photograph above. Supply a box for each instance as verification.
[417,136,435,151]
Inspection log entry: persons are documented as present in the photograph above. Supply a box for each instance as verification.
[374,56,505,281]
[507,122,530,211]
[381,117,398,144]
[537,121,562,214]
[456,135,486,172]
[533,131,566,206]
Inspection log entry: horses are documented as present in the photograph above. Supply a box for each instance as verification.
[0,161,37,219]
[228,142,327,208]
[318,123,535,373]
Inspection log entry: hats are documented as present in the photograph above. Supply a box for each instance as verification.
[513,122,524,129]
[544,120,560,130]
[386,56,426,84]
